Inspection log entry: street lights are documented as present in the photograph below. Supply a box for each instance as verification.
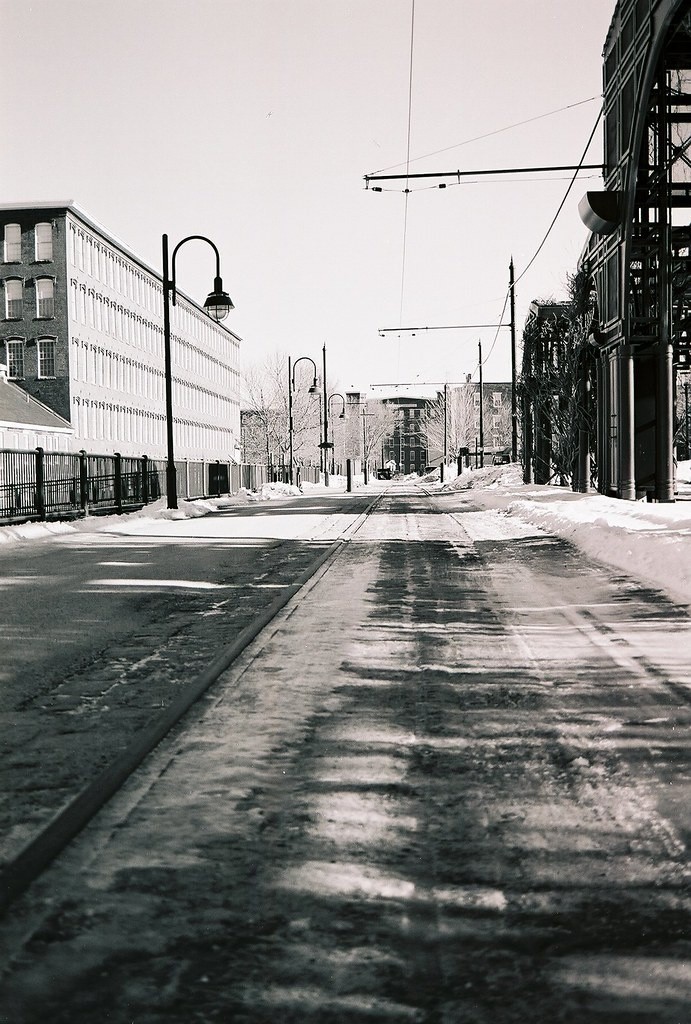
[286,355,320,487]
[320,342,347,485]
[162,232,235,509]
